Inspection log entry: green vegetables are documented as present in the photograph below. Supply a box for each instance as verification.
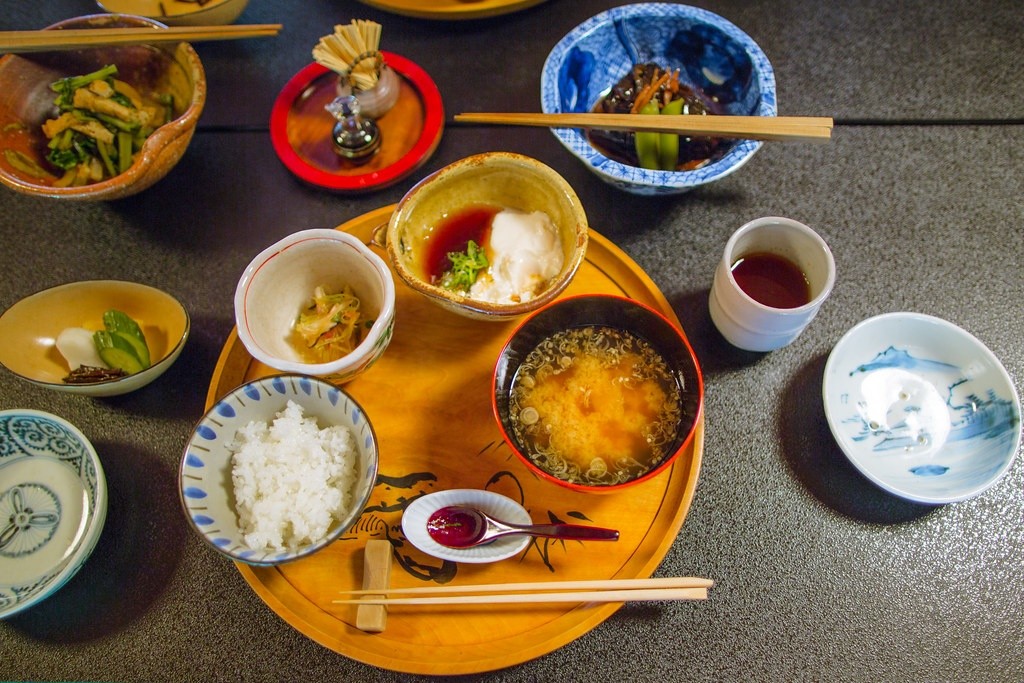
[442,239,488,290]
[4,65,177,184]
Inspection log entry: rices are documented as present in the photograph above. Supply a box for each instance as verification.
[224,397,360,551]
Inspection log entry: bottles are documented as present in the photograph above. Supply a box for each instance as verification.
[324,95,382,165]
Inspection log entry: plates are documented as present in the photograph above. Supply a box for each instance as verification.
[361,1,540,21]
[823,311,1022,504]
[401,488,532,563]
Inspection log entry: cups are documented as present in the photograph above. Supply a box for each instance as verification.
[708,216,836,352]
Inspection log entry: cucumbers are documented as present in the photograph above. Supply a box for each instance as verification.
[93,309,151,375]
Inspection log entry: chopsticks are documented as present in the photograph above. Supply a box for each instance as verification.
[1,24,285,54]
[332,577,714,604]
[453,113,834,140]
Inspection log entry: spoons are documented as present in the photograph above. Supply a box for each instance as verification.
[426,506,620,549]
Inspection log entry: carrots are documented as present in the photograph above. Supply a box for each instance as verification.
[630,66,679,114]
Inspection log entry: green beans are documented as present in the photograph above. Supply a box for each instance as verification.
[633,97,687,171]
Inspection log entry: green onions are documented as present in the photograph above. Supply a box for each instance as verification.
[510,327,683,485]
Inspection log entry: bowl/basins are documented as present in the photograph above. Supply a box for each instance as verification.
[0,13,206,203]
[234,229,394,385]
[0,409,107,619]
[490,294,704,495]
[95,1,247,29]
[370,152,590,322]
[178,374,379,566]
[0,279,191,397]
[540,3,778,195]
